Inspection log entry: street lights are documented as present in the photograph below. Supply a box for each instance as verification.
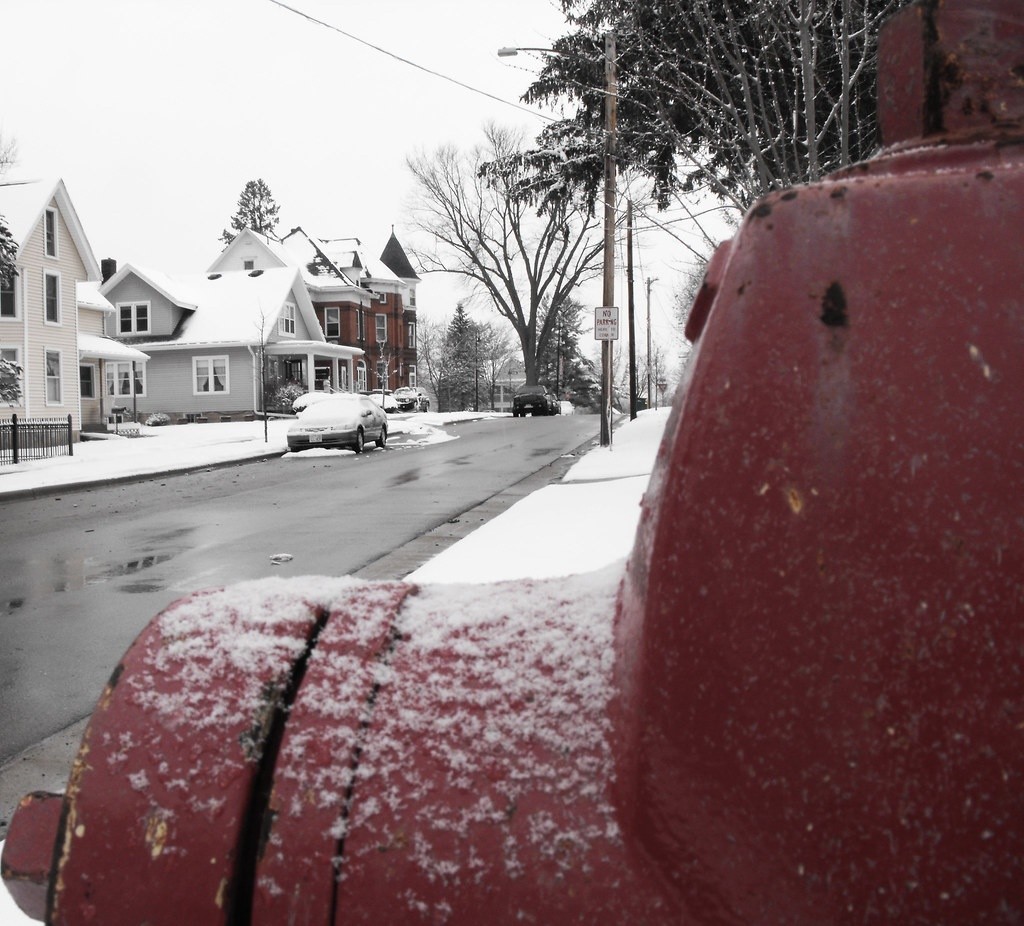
[497,33,618,443]
[644,277,659,409]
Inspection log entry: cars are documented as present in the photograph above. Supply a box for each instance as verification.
[561,401,575,417]
[394,387,431,412]
[512,385,562,417]
[359,389,398,413]
[287,394,388,454]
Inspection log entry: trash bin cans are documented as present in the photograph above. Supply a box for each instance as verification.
[637,398,647,410]
